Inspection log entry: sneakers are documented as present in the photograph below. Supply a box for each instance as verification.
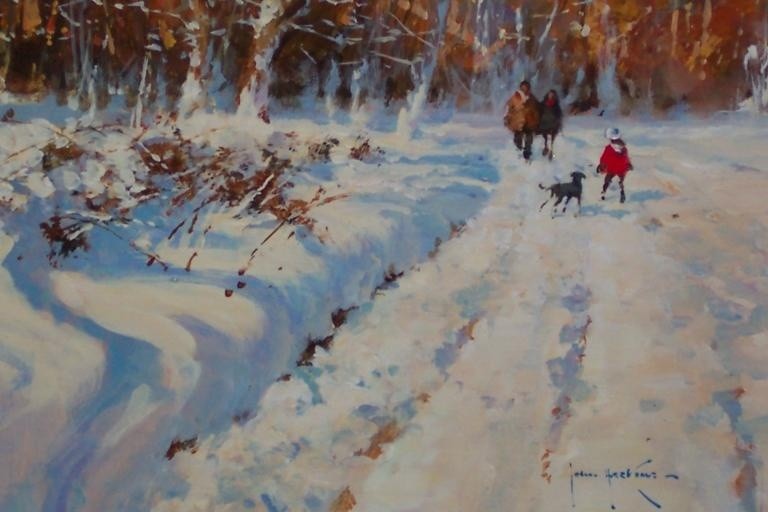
[600,190,626,203]
[516,146,554,161]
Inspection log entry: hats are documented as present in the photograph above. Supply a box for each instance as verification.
[606,126,622,140]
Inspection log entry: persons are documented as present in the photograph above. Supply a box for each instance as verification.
[596,127,634,205]
[538,90,565,160]
[504,80,538,159]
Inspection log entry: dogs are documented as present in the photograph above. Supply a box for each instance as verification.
[539,172,585,219]
